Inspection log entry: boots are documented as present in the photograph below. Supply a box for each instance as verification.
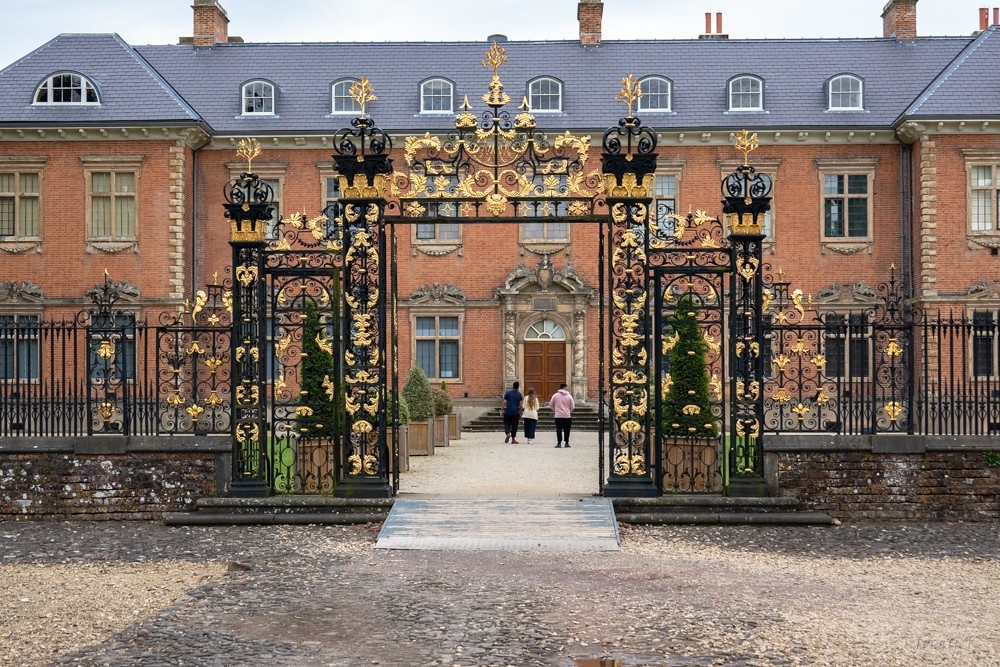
[505,434,510,443]
[512,438,518,444]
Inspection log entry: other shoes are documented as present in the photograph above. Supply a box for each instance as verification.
[565,444,570,447]
[555,444,561,448]
[528,439,532,443]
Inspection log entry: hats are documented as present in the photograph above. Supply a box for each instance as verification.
[560,384,568,388]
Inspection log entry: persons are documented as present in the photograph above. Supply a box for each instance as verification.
[549,384,574,447]
[522,387,539,444]
[500,382,523,444]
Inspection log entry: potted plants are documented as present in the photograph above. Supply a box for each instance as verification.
[615,296,722,490]
[293,299,462,489]
